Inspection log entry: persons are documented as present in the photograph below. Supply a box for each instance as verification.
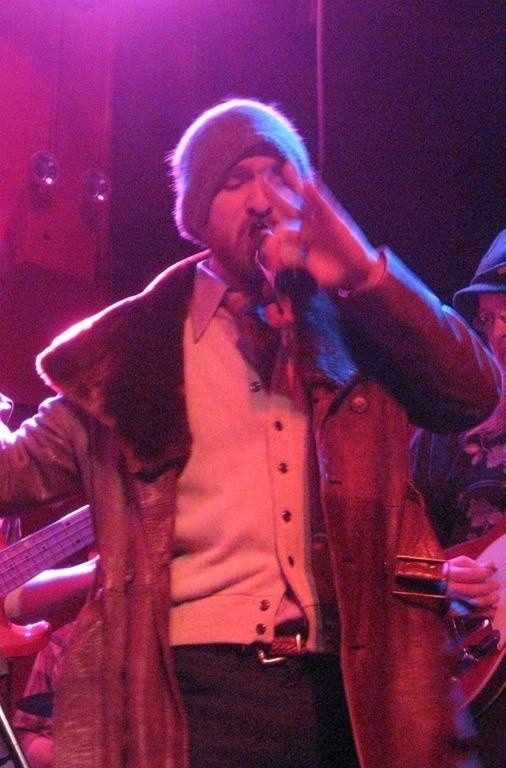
[0,390,99,624]
[11,542,100,768]
[406,228,506,768]
[0,99,502,768]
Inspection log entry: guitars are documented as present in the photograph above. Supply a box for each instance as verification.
[445,511,506,722]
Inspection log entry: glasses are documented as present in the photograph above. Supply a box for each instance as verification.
[473,306,505,333]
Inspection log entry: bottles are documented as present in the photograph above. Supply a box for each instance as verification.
[243,218,318,310]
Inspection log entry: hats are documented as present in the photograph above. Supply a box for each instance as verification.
[452,230,506,318]
[174,99,312,245]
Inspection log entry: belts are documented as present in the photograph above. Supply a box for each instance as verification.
[254,624,309,665]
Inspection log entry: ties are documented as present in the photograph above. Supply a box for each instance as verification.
[222,288,281,395]
